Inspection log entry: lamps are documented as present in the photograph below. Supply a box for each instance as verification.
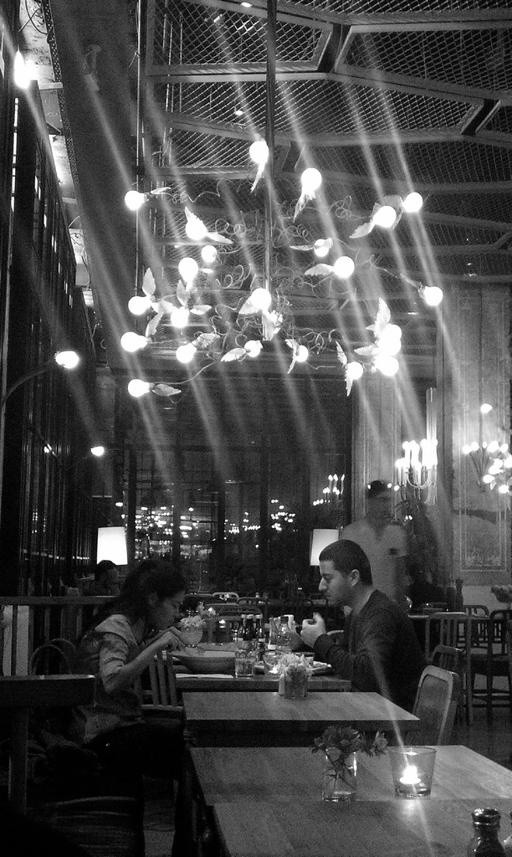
[114,1,447,397]
[98,526,132,571]
[395,437,439,502]
[465,401,511,494]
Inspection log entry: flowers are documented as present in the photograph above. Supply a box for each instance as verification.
[313,725,386,800]
[274,653,312,699]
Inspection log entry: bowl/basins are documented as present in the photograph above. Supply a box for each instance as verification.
[170,648,237,672]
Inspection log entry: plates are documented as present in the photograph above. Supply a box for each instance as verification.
[262,659,330,674]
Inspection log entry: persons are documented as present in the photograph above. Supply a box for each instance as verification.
[302,540,425,708]
[339,482,408,606]
[84,560,121,626]
[77,563,194,772]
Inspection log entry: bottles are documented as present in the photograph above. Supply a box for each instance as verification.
[274,616,294,663]
[255,612,270,653]
[239,615,250,653]
[471,808,503,844]
[241,615,258,655]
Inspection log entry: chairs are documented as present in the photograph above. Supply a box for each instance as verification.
[237,597,271,616]
[464,605,489,642]
[426,612,474,725]
[0,673,145,857]
[29,644,69,674]
[282,598,313,617]
[408,666,461,745]
[472,609,512,731]
[49,639,75,661]
[140,646,184,718]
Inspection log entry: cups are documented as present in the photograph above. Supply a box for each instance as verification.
[387,747,437,798]
[284,670,309,699]
[323,747,358,803]
